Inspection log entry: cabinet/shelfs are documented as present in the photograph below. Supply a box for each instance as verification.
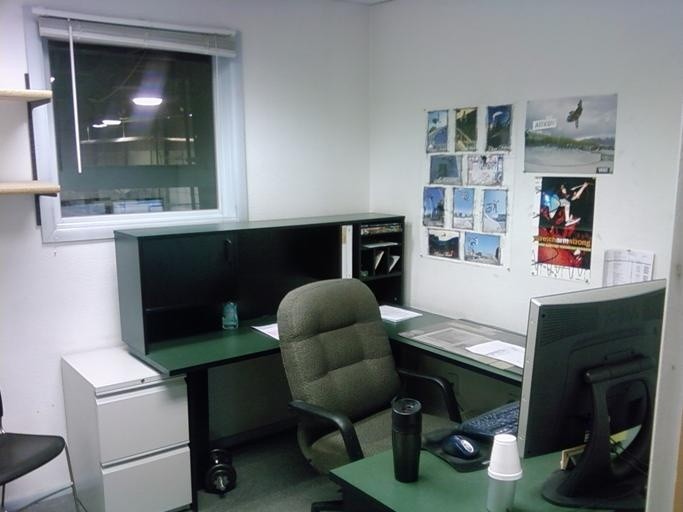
[391,397,422,483]
[0,89,61,197]
[61,344,195,511]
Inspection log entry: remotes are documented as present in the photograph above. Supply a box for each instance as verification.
[441,435,481,459]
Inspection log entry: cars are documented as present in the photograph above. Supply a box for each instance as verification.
[487,433,523,481]
[486,472,517,512]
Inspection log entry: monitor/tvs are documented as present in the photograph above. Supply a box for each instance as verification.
[360,227,402,236]
[360,221,401,228]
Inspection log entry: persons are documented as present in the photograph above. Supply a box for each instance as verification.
[425,107,509,263]
[556,182,580,226]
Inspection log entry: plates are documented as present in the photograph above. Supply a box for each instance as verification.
[0,89,61,197]
[61,344,195,511]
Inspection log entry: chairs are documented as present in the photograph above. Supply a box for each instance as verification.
[0,390,76,511]
[277,278,461,510]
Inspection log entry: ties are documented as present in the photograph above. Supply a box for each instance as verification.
[516,278,668,508]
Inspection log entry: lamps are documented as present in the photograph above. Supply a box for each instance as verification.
[277,278,461,510]
[0,390,76,511]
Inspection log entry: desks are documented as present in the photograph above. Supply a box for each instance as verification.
[125,306,385,497]
[384,308,527,386]
[330,425,642,509]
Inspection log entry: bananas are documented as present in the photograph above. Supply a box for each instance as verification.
[459,399,521,443]
